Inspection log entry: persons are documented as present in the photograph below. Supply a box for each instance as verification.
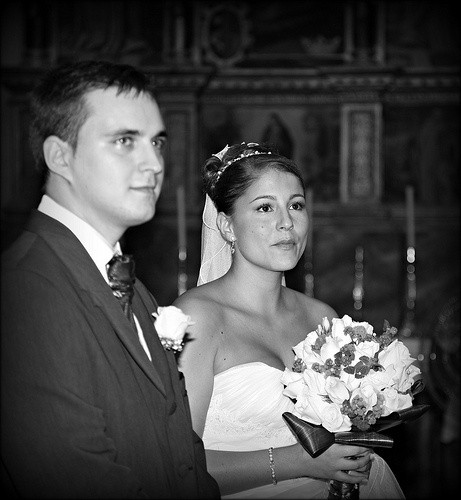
[175,140,406,500]
[0,55,220,500]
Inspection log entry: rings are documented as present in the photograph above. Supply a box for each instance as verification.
[348,470,352,475]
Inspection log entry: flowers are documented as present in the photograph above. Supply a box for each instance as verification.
[281,314,429,500]
[151,305,196,353]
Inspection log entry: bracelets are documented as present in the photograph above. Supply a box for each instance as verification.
[269,447,277,485]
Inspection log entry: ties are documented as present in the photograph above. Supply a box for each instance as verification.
[106,254,139,341]
